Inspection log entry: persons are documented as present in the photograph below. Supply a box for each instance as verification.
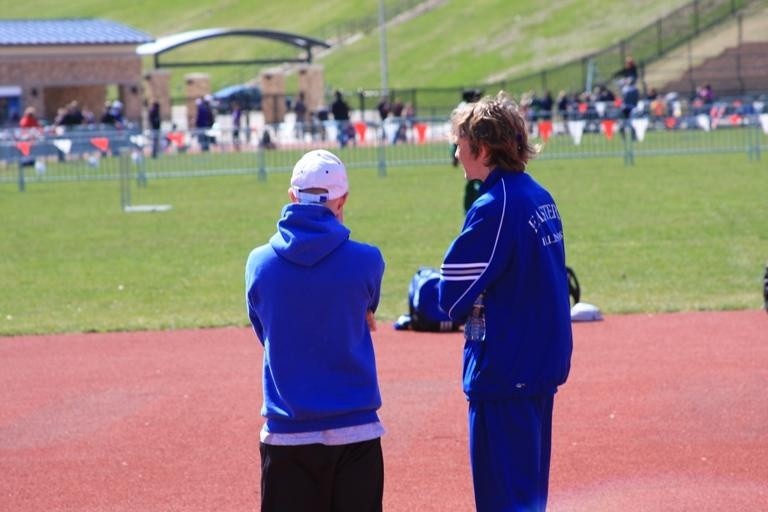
[407,89,572,512]
[245,149,385,512]
[8,56,768,160]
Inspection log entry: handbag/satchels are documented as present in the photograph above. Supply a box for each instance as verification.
[393,268,458,332]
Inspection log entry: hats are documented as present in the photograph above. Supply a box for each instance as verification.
[290,149,348,203]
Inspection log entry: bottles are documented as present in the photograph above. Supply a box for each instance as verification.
[464,293,486,342]
